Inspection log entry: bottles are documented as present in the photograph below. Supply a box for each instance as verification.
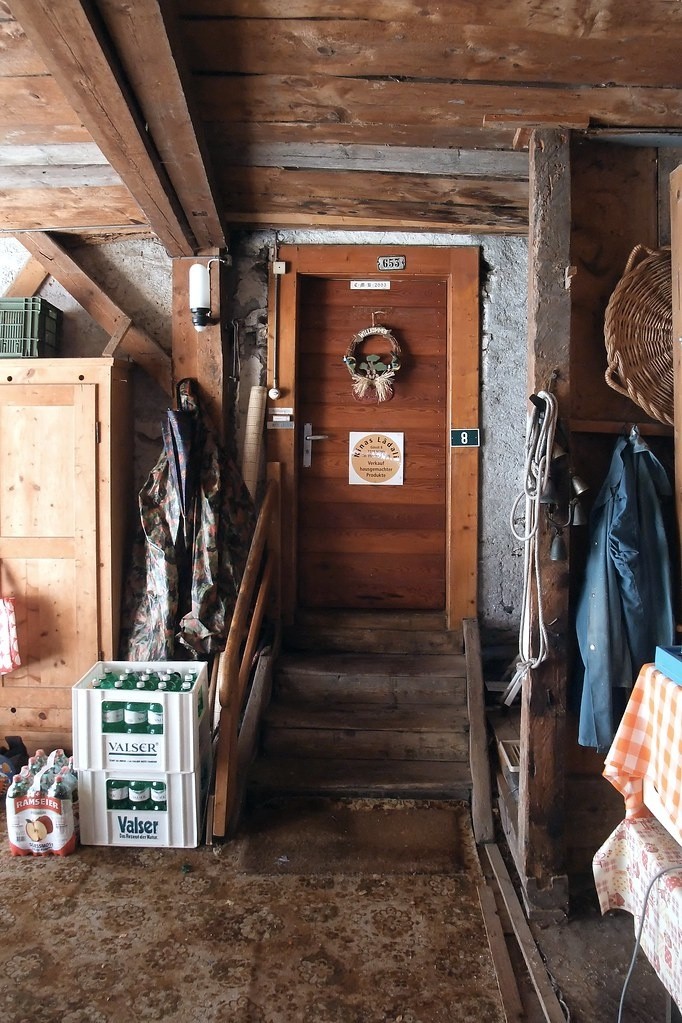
[93,668,202,810]
[5,748,79,857]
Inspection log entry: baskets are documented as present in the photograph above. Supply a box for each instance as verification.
[0,297,63,358]
[605,243,674,428]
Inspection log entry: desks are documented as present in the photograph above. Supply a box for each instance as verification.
[590,663,682,1023]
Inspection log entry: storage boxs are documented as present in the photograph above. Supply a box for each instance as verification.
[70,660,211,771]
[0,296,64,359]
[76,749,215,849]
[655,646,682,686]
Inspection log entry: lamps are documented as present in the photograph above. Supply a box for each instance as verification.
[188,263,220,332]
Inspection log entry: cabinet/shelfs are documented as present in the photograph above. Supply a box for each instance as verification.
[0,356,135,758]
